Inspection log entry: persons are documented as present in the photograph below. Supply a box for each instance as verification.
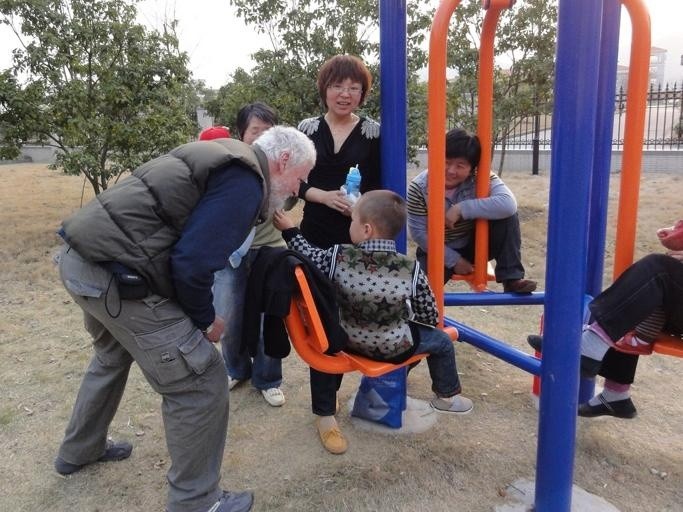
[527,221,683,419]
[407,129,538,375]
[613,220,683,355]
[54,126,318,511]
[211,103,299,407]
[295,54,380,455]
[274,189,474,414]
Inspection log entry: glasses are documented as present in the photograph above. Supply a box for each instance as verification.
[327,84,363,93]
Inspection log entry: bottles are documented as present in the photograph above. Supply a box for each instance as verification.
[344,164,362,197]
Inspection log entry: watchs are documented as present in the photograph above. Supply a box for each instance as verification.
[202,321,215,336]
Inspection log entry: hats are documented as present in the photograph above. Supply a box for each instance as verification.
[199,127,230,141]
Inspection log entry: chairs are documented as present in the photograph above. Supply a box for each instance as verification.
[282,255,460,379]
[446,258,499,282]
[653,331,683,359]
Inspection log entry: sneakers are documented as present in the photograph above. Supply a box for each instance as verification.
[228,374,240,391]
[612,332,654,358]
[54,440,133,475]
[526,333,602,379]
[315,414,349,455]
[429,395,474,415]
[503,278,538,293]
[162,490,254,512]
[260,388,286,407]
[335,391,341,416]
[578,398,637,419]
